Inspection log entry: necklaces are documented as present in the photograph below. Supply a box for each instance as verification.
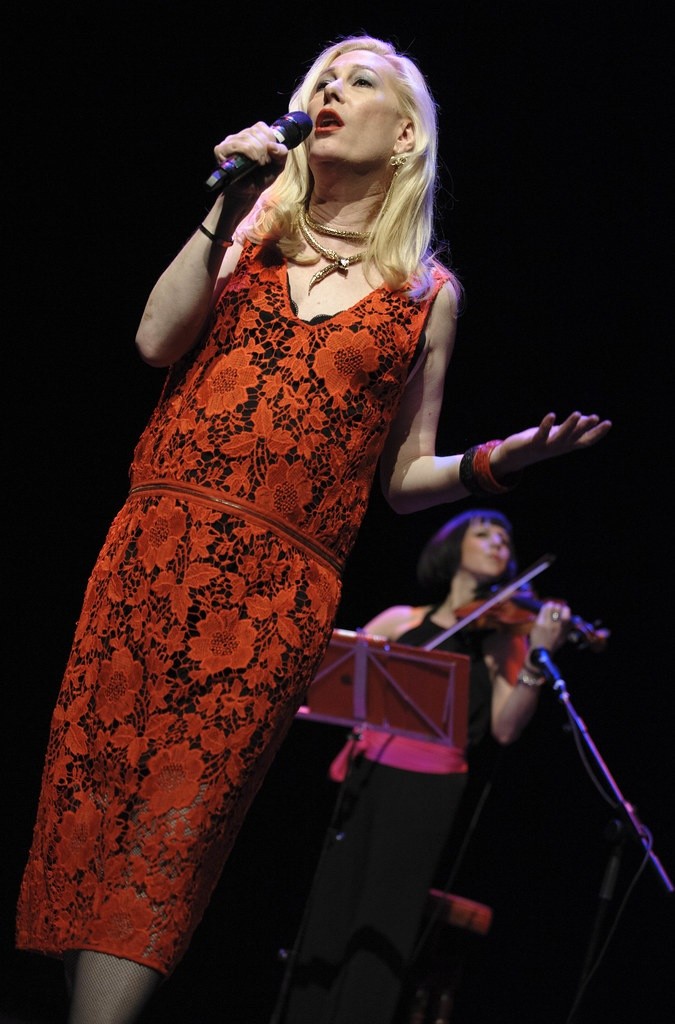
[299,212,367,291]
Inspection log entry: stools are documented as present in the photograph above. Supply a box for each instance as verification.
[407,887,492,1024]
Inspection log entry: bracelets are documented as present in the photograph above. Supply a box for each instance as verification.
[198,223,233,247]
[522,663,543,677]
[459,439,508,506]
[518,674,546,687]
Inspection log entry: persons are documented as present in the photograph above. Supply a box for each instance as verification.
[271,511,571,1024]
[15,34,612,1024]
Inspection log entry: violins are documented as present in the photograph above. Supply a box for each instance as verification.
[451,575,614,651]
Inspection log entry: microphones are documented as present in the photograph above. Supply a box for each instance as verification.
[204,107,312,197]
[528,646,571,705]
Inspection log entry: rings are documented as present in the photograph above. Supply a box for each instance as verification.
[551,611,560,622]
